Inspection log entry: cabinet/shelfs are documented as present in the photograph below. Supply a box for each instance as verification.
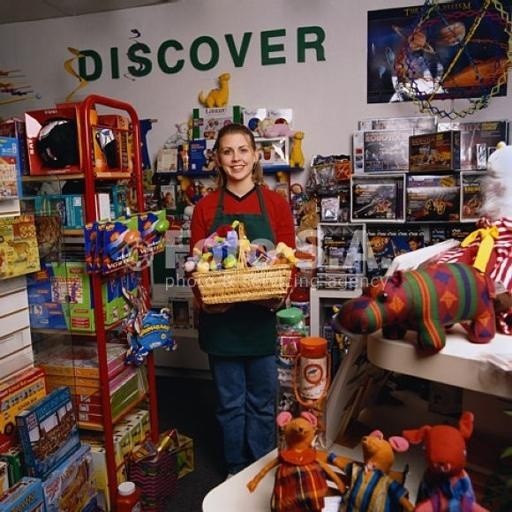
[0,94,304,512]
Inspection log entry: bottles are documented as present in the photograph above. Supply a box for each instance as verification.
[115,481,146,512]
[274,308,331,455]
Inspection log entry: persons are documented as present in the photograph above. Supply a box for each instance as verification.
[188,123,299,480]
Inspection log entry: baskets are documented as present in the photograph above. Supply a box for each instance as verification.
[184,264,292,305]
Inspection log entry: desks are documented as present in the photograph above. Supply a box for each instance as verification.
[366,319,512,438]
